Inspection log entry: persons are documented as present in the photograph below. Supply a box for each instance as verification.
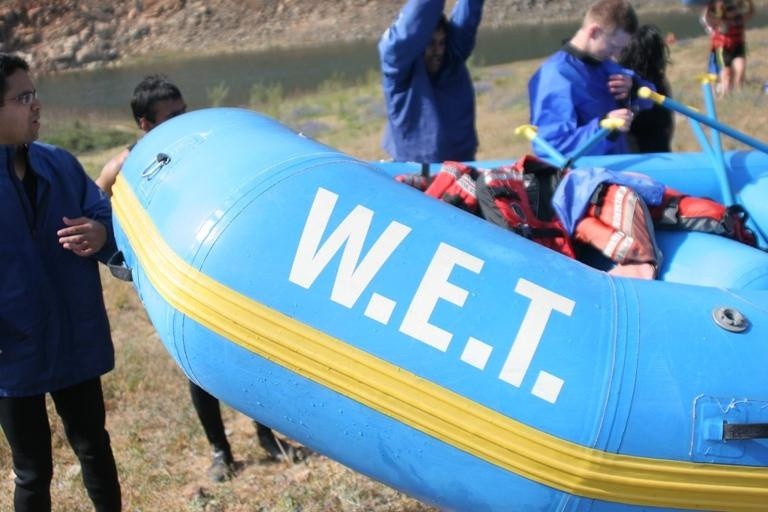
[612,22,676,156]
[92,74,308,484]
[374,0,484,166]
[0,51,120,512]
[700,0,755,96]
[526,1,659,160]
[697,2,729,95]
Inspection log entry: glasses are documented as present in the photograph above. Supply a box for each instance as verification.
[5,90,39,107]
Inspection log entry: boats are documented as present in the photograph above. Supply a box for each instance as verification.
[110,108,768,512]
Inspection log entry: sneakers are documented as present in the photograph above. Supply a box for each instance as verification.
[208,437,303,480]
